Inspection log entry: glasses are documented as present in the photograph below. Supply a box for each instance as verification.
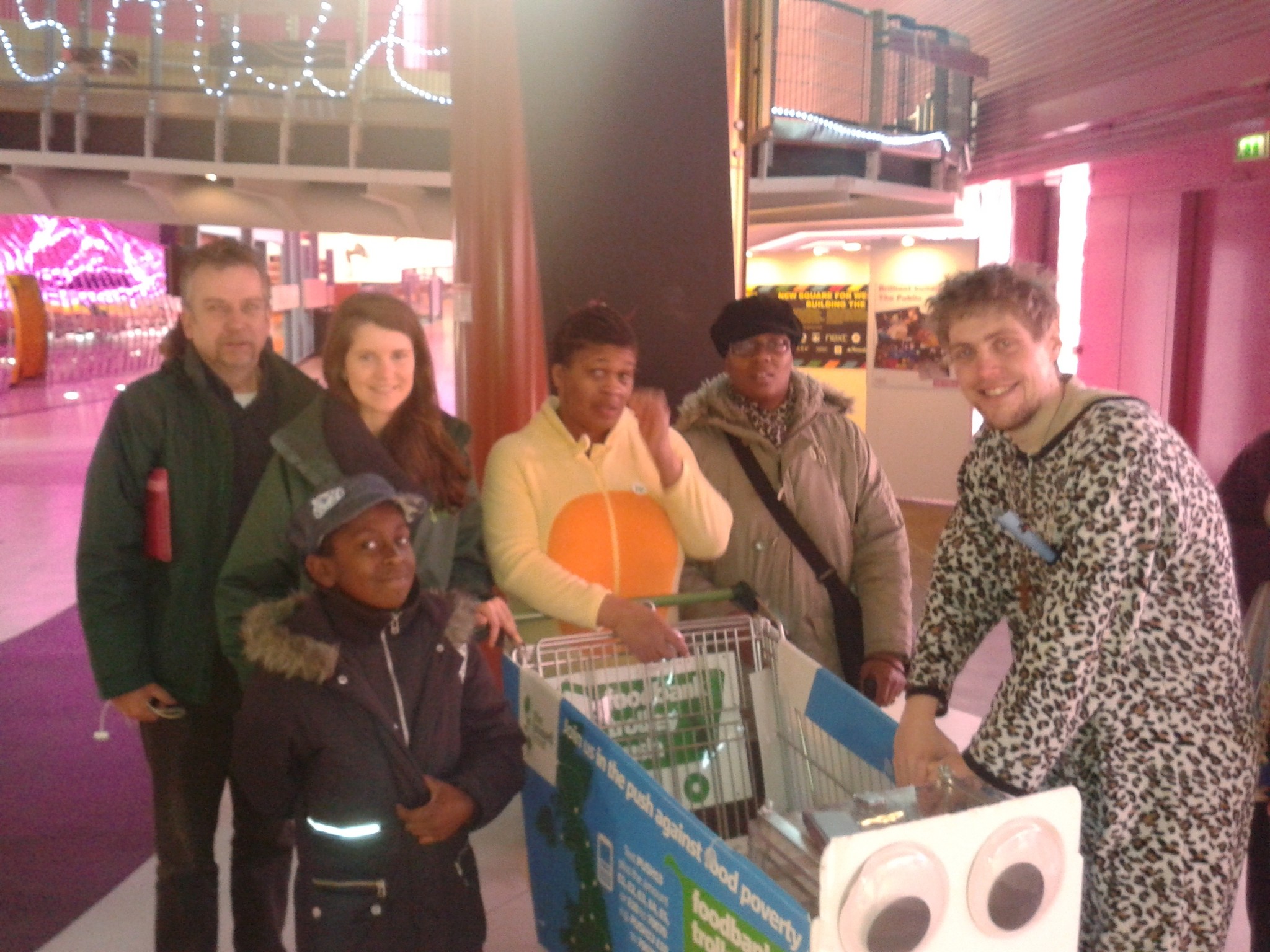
[721,339,792,357]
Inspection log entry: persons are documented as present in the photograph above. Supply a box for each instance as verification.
[662,293,916,706]
[224,470,531,952]
[1206,428,1270,952]
[68,238,322,952]
[214,291,524,702]
[478,297,735,665]
[887,254,1259,952]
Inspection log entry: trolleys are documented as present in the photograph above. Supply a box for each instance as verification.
[473,578,1088,952]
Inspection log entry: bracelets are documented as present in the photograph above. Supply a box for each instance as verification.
[864,657,907,675]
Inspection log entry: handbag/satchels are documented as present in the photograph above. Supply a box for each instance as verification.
[303,879,390,952]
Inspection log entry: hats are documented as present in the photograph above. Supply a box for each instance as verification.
[289,472,409,554]
[711,293,804,356]
